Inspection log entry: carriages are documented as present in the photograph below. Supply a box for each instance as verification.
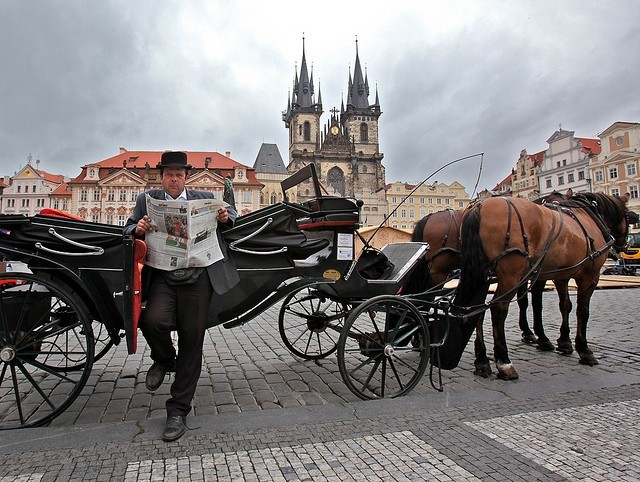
[0,162,631,431]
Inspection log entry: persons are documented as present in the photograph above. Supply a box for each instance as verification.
[121,150,239,442]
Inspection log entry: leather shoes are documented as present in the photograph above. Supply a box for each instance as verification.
[145,363,167,390]
[162,414,187,442]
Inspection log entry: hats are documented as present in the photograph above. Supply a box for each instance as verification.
[155,152,192,169]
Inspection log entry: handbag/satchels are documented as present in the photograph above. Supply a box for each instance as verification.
[165,268,204,285]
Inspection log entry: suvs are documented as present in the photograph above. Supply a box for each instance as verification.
[619,228,640,264]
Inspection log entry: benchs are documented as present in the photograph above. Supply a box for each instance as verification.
[280,163,362,230]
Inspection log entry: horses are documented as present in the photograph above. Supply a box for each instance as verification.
[411,188,573,351]
[455,191,631,379]
